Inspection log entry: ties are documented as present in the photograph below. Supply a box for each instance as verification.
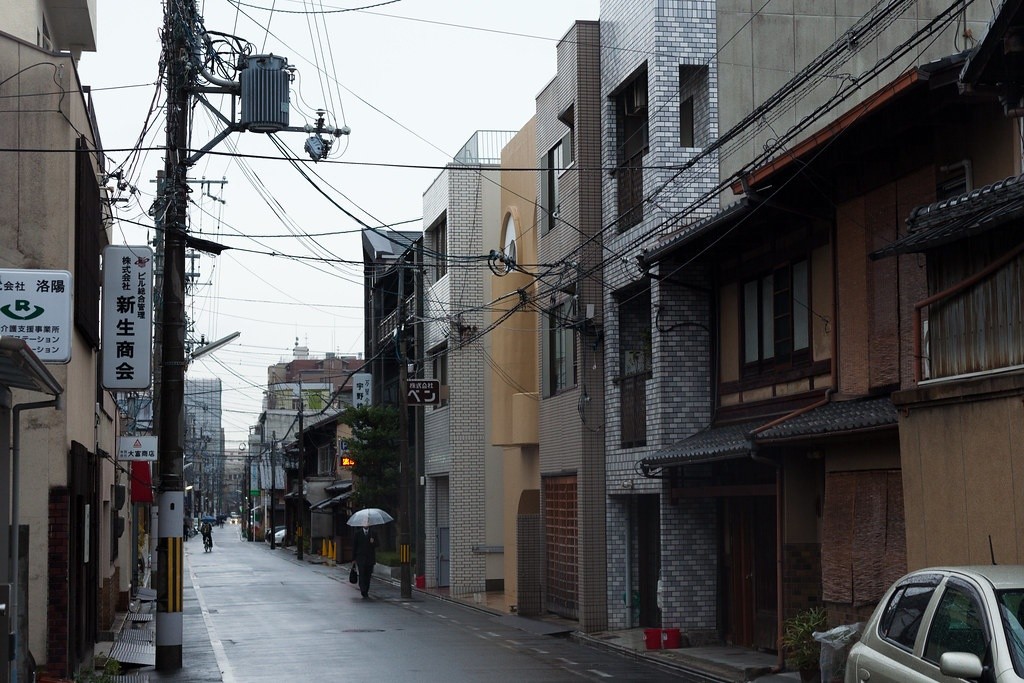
[366,531,367,533]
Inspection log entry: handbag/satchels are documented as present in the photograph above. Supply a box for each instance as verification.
[349,563,357,584]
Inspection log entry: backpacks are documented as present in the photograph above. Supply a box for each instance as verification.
[203,523,210,534]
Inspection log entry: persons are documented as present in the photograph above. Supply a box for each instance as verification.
[201,520,212,549]
[352,519,377,598]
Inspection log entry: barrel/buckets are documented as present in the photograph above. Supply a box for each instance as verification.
[643,629,661,649]
[416,576,425,587]
[663,629,681,649]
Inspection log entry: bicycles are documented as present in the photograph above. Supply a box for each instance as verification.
[203,530,214,553]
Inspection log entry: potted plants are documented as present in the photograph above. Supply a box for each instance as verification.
[779,605,832,683]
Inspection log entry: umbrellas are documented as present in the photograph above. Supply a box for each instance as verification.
[200,516,216,521]
[347,509,393,538]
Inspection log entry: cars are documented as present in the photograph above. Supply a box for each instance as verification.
[844,564,1024,683]
[266,525,285,545]
